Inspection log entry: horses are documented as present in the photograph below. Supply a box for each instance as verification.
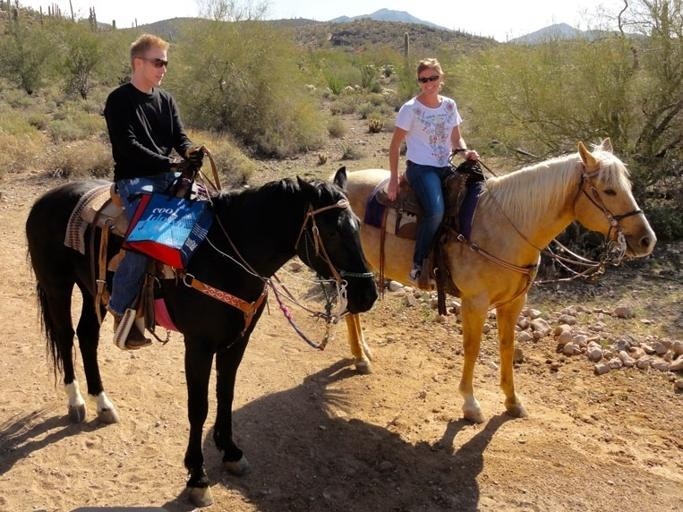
[324,138,656,423]
[27,168,378,508]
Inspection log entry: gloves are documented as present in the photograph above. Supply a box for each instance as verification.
[188,146,203,168]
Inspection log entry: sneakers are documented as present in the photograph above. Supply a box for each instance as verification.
[410,260,423,279]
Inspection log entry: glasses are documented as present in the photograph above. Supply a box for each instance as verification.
[419,76,441,82]
[140,56,169,68]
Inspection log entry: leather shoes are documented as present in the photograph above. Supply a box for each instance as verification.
[108,306,152,350]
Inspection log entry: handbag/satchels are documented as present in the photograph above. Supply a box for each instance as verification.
[126,172,214,269]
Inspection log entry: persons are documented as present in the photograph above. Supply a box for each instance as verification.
[106,36,203,346]
[390,57,480,281]
